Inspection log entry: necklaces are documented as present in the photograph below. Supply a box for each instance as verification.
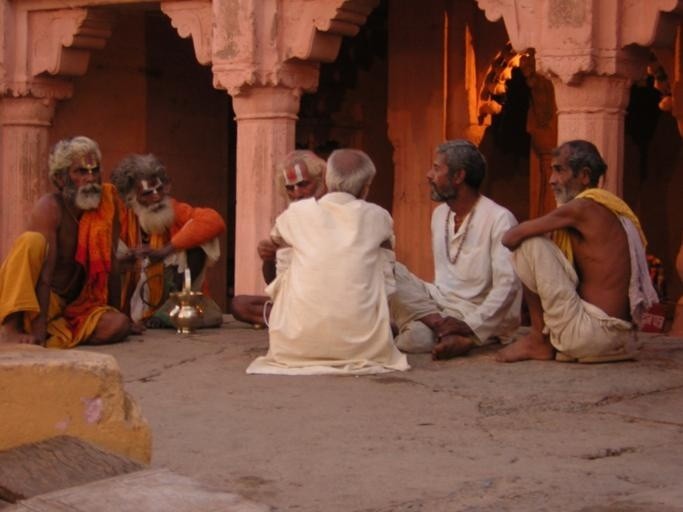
[445,198,479,264]
[65,202,79,225]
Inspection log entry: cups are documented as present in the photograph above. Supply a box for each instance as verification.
[168,292,204,335]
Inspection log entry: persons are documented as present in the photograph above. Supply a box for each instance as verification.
[111,152,226,330]
[246,148,412,376]
[0,136,146,348]
[388,139,523,360]
[229,149,326,329]
[493,140,640,362]
